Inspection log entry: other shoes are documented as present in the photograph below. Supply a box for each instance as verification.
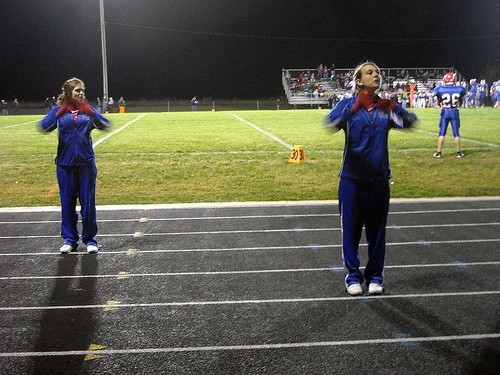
[345,282,363,296]
[87,245,98,252]
[432,152,441,157]
[457,152,464,158]
[369,281,383,294]
[59,244,74,252]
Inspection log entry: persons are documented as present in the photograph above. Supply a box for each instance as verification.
[40,78,109,252]
[285,64,500,110]
[0,100,8,116]
[118,97,125,114]
[13,99,19,115]
[433,72,466,159]
[45,96,113,114]
[191,97,199,112]
[324,62,417,294]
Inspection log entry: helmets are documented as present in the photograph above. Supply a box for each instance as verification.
[443,72,456,86]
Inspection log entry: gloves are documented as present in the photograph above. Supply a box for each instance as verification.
[55,100,73,118]
[373,99,395,111]
[352,91,369,111]
[76,102,94,115]
